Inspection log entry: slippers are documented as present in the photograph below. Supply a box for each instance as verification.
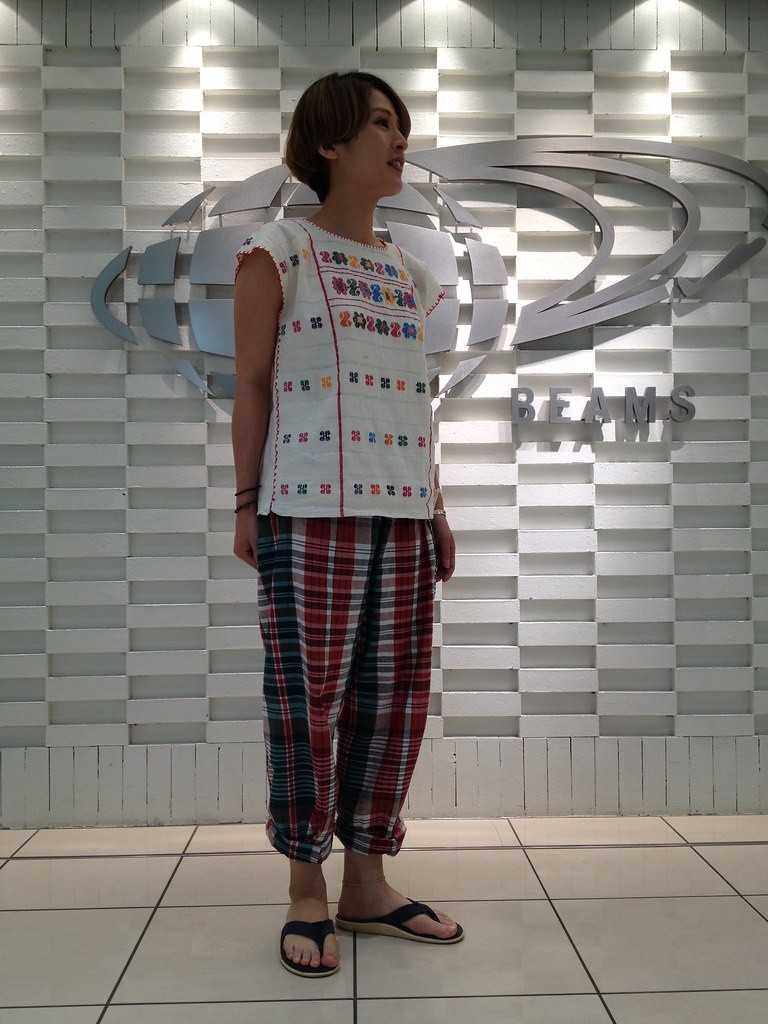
[280,918,340,978]
[335,898,464,944]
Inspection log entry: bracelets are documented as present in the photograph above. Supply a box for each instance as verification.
[432,509,446,516]
[234,499,257,513]
[235,485,260,497]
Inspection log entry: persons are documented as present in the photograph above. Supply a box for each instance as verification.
[230,72,464,978]
